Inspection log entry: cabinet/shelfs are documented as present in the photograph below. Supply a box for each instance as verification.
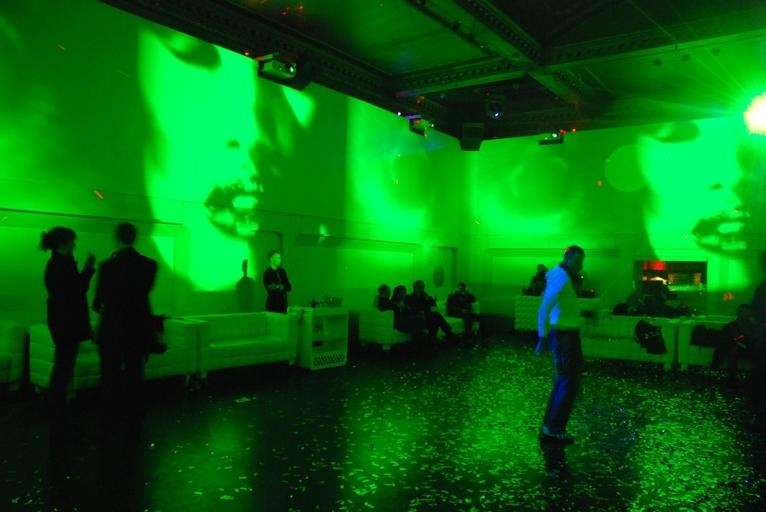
[299,303,351,372]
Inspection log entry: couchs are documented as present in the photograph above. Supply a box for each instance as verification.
[26,314,201,391]
[514,301,742,373]
[359,301,485,349]
[189,309,299,370]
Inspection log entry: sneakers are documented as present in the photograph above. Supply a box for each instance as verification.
[542,425,572,440]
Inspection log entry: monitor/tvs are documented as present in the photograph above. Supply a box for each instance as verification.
[633,260,710,316]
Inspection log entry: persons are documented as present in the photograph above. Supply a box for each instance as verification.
[261,250,292,315]
[609,293,647,317]
[631,84,766,294]
[404,280,461,356]
[531,244,586,443]
[446,282,479,343]
[88,222,159,452]
[376,283,416,360]
[641,288,675,318]
[38,226,98,432]
[124,24,325,300]
[705,302,758,378]
[524,263,548,296]
[390,284,441,363]
